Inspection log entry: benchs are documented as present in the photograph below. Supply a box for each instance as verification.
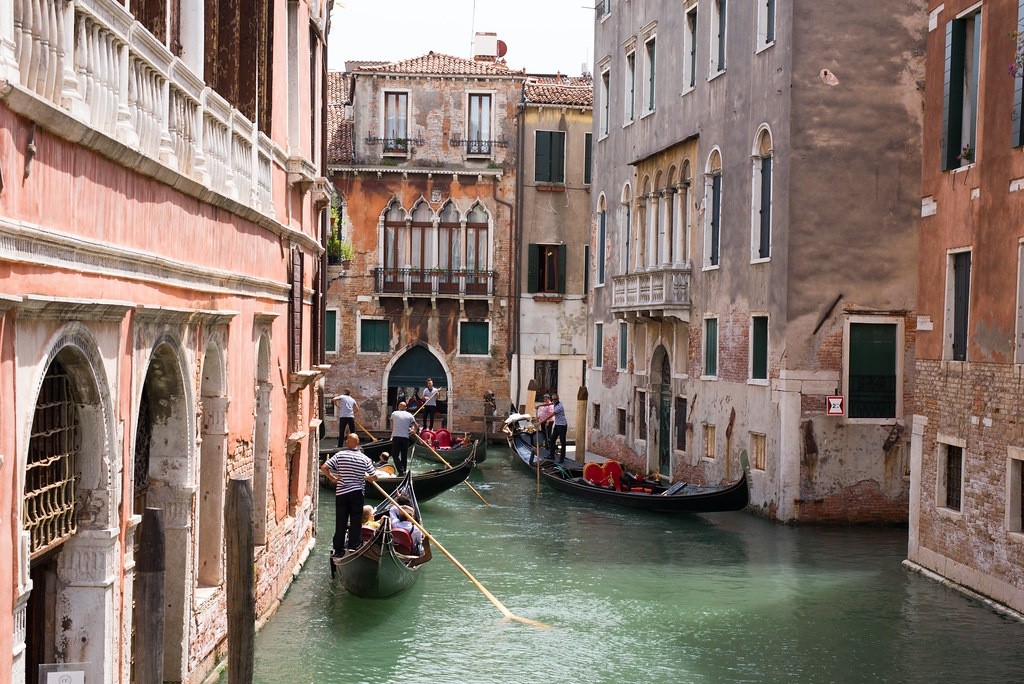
[660,481,686,496]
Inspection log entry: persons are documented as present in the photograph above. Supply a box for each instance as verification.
[534,393,568,464]
[423,378,439,431]
[333,388,359,448]
[321,433,423,559]
[390,402,418,477]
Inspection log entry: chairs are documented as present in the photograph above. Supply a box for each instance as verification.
[362,525,376,541]
[391,528,413,555]
[436,429,453,449]
[419,430,439,448]
[602,460,625,490]
[582,463,607,486]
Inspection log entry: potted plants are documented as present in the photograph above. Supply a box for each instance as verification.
[385,267,401,276]
[340,241,356,270]
[409,266,423,276]
[429,266,446,276]
[328,232,341,266]
[453,268,469,277]
[330,208,340,230]
[473,269,489,278]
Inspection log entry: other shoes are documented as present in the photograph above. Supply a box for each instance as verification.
[334,445,343,448]
[397,472,407,477]
[544,456,555,460]
[557,461,564,464]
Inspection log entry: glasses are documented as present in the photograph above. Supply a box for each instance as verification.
[552,398,558,400]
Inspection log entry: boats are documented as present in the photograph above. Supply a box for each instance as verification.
[411,432,487,465]
[333,469,423,599]
[318,425,419,469]
[318,439,478,504]
[502,402,751,511]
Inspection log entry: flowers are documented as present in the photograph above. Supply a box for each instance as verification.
[1000,30,1024,79]
[956,144,975,161]
